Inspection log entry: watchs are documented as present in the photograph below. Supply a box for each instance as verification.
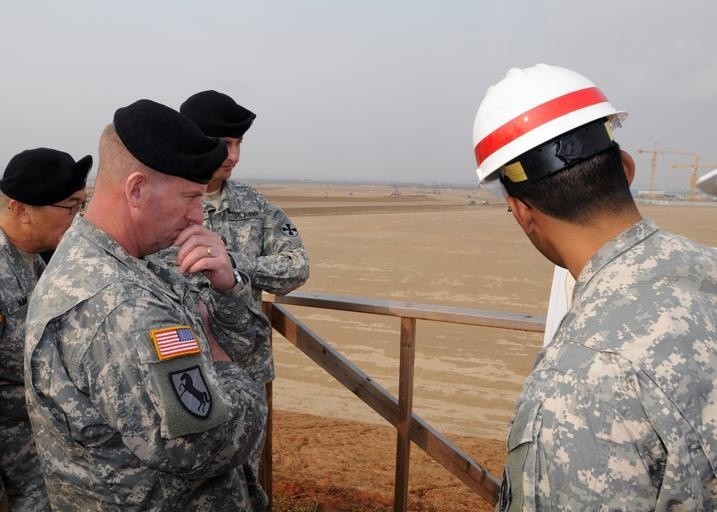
[207,271,245,296]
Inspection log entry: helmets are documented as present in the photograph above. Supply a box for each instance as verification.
[469,63,633,189]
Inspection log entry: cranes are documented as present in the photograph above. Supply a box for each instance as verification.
[635,137,692,198]
[669,154,717,196]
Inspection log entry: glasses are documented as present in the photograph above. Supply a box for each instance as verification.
[51,199,89,217]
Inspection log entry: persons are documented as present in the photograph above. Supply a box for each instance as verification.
[22,98,273,511]
[471,63,717,512]
[144,88,310,390]
[0,146,94,512]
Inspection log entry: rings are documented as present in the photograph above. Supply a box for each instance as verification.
[207,247,211,258]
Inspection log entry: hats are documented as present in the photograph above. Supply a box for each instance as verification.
[179,90,257,140]
[1,147,92,207]
[114,99,230,184]
